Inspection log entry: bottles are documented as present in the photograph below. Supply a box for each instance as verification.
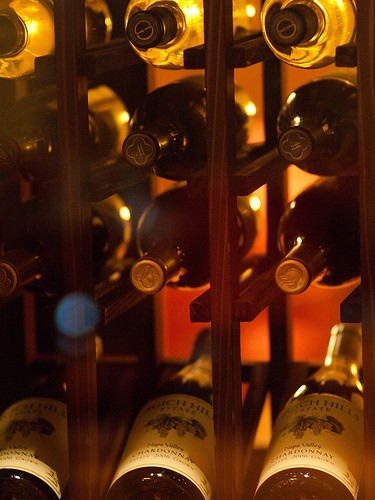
[260,0,357,68]
[105,330,217,500]
[130,185,257,294]
[273,72,360,176]
[0,338,68,500]
[121,74,256,181]
[275,176,360,294]
[0,85,141,168]
[0,193,132,298]
[125,0,263,71]
[254,324,363,500]
[0,0,111,79]
